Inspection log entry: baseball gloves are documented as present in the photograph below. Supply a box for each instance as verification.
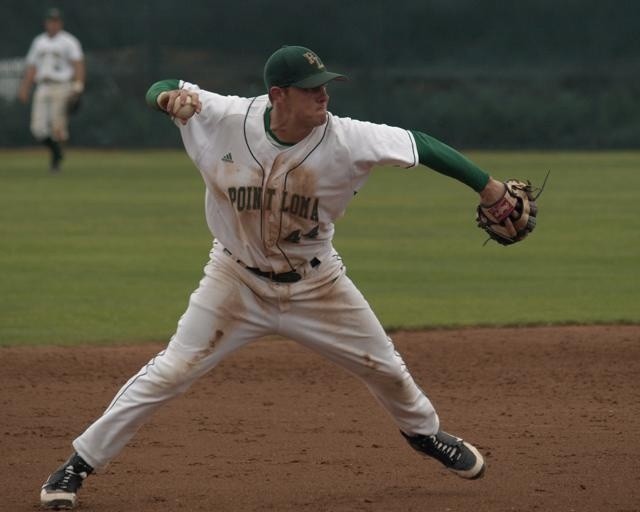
[67,88,83,115]
[475,179,539,243]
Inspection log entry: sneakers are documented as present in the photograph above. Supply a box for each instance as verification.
[40,452,94,509]
[398,428,486,481]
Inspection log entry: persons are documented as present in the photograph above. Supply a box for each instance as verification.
[38,45,538,509]
[18,8,86,169]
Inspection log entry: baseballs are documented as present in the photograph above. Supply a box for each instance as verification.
[172,95,196,119]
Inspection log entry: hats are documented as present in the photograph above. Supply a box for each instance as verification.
[263,45,347,93]
